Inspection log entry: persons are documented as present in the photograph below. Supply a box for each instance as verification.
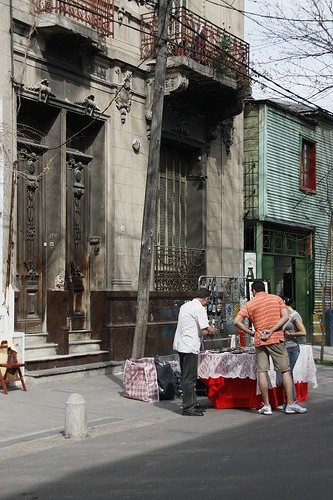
[278,294,307,411]
[234,280,308,415]
[174,289,219,417]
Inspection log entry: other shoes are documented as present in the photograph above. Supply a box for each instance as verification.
[194,403,209,411]
[182,407,203,416]
[277,403,287,411]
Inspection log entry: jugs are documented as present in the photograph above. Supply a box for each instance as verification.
[228,334,237,350]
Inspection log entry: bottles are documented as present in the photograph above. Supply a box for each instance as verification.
[207,279,224,337]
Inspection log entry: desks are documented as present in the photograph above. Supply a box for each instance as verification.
[198,345,318,410]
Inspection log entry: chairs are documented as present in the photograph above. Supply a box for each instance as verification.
[0,340,27,394]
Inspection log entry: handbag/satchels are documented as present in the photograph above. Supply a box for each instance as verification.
[120,357,158,402]
[155,359,175,400]
[169,360,197,398]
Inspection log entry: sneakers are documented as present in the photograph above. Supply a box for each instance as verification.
[258,401,273,415]
[285,401,307,413]
